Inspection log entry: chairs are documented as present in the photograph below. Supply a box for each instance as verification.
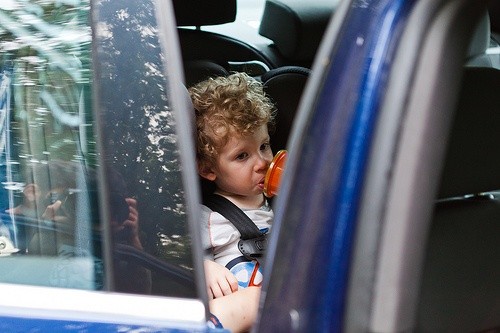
[12,0,500,333]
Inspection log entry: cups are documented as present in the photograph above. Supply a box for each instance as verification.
[263,149,286,197]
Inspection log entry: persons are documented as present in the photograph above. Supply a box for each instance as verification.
[189,72,277,333]
[3,163,159,295]
